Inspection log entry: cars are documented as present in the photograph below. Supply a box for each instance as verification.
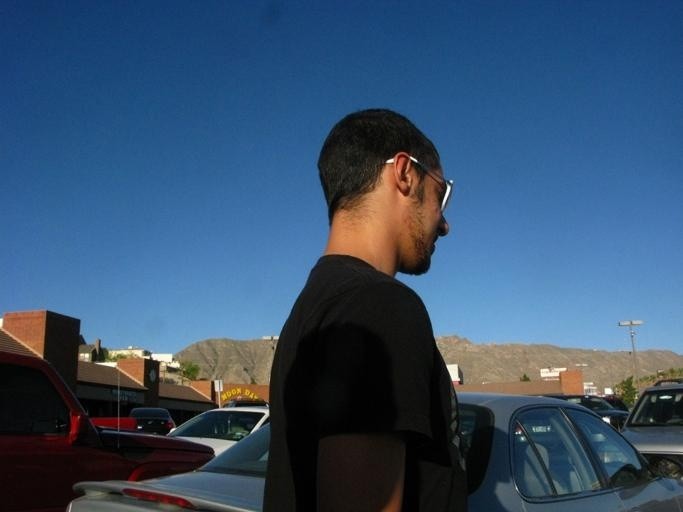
[151,404,272,462]
[603,395,629,412]
[131,406,174,436]
[59,391,682,512]
[546,394,628,430]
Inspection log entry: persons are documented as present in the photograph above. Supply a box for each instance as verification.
[263,108,467,510]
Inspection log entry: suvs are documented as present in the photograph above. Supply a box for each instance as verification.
[0,349,212,512]
[618,378,682,482]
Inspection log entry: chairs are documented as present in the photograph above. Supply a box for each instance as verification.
[512,442,567,497]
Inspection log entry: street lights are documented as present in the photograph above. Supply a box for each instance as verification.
[262,335,279,357]
[617,318,644,382]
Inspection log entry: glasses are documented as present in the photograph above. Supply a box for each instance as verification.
[382,149,456,214]
